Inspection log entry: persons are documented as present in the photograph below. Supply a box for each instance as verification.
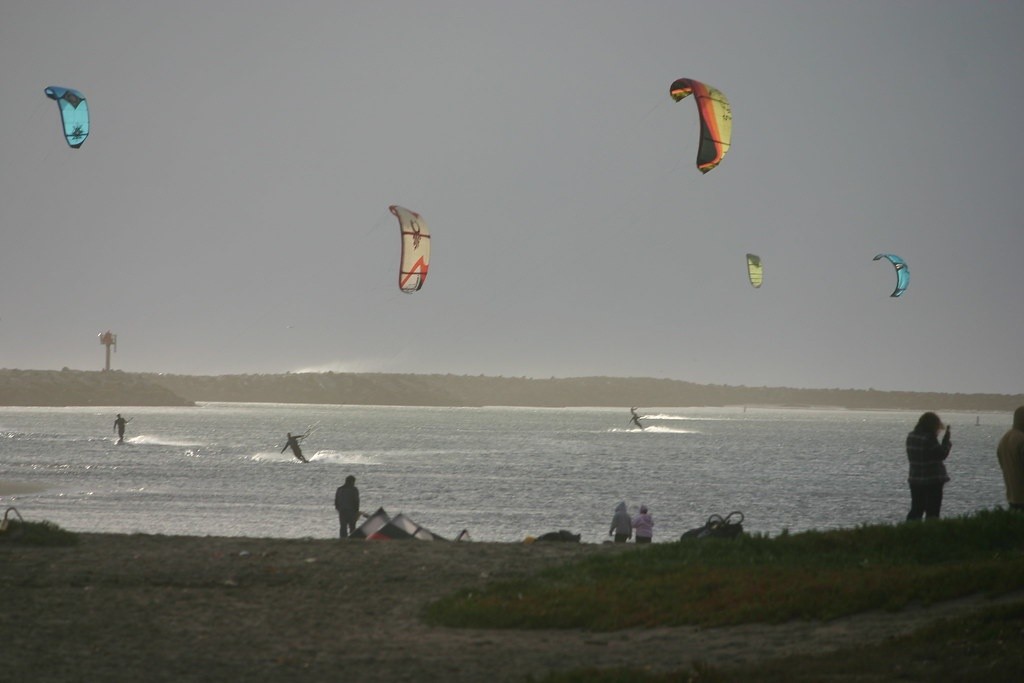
[113,413,131,442]
[906,412,952,521]
[630,406,643,429]
[335,475,360,539]
[609,501,654,543]
[281,433,308,463]
[997,406,1024,511]
